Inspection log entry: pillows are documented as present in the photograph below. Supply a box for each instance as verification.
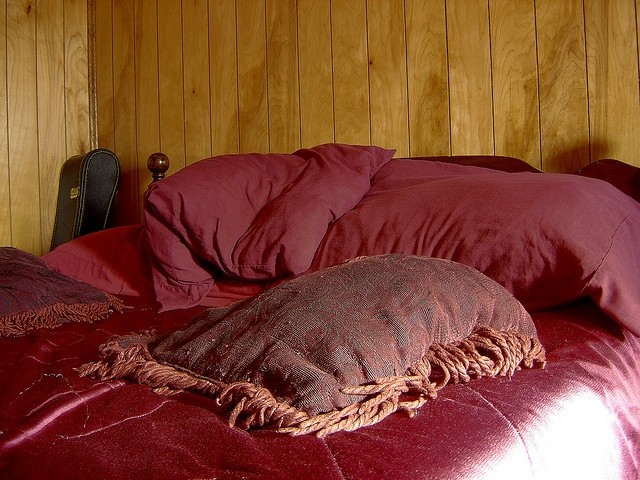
[76,254,545,438]
[143,143,396,313]
[37,223,293,309]
[308,159,640,334]
[1,245,134,339]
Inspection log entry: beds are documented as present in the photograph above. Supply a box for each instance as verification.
[1,142,640,479]
